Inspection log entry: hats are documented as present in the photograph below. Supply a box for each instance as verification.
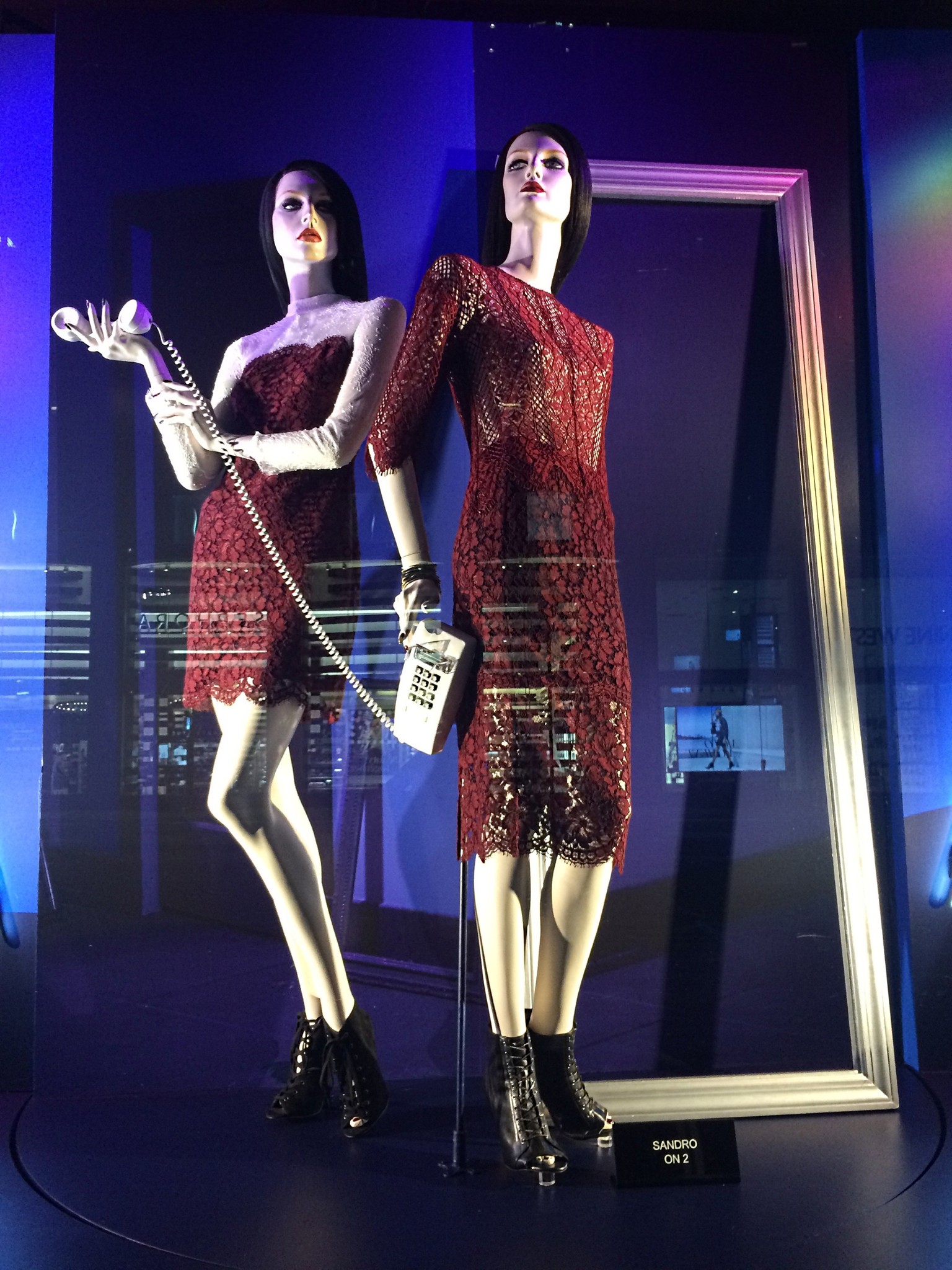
[715,708,722,713]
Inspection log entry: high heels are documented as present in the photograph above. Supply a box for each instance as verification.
[480,1027,569,1185]
[729,761,734,769]
[265,1011,324,1121]
[324,999,390,1138]
[705,762,715,769]
[529,1021,617,1140]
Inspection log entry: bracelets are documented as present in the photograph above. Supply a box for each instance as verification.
[400,563,441,591]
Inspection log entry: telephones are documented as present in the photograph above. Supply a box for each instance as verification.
[50,303,153,343]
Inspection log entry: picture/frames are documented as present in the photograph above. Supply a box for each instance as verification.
[524,160,900,1122]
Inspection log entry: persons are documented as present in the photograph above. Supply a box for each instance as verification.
[706,708,734,770]
[64,162,407,1138]
[368,125,633,1185]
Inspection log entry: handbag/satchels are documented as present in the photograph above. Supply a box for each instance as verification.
[711,728,716,734]
